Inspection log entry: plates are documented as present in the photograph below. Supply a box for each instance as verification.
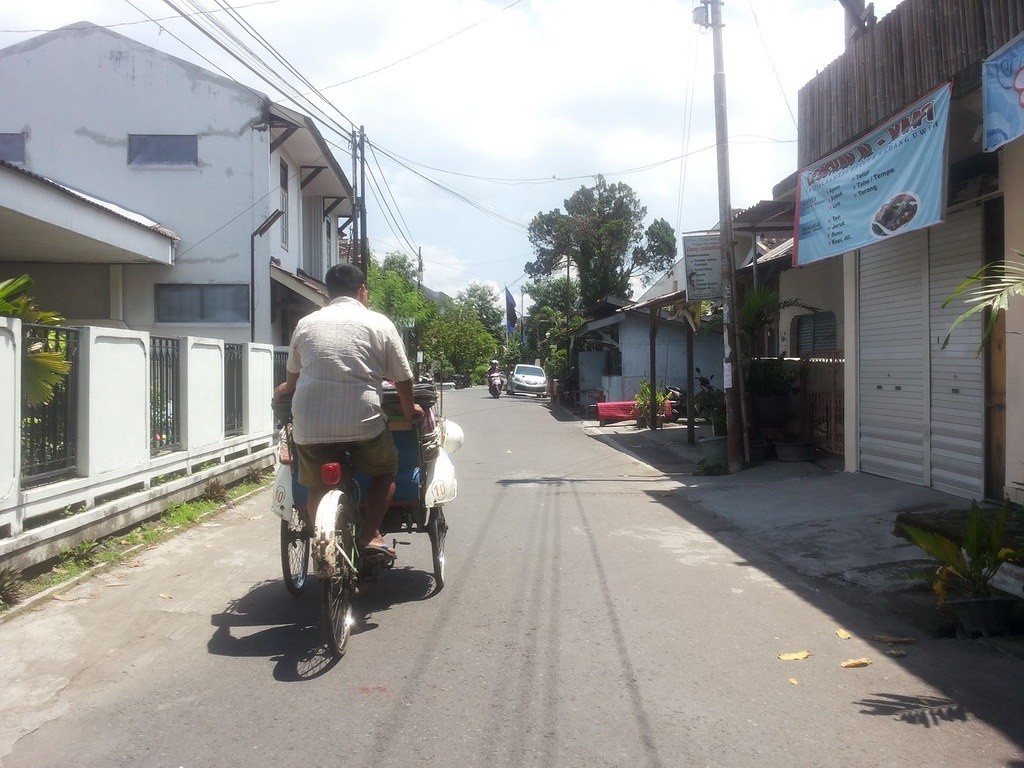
[868,191,921,238]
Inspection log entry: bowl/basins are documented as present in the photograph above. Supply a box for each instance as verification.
[772,438,820,461]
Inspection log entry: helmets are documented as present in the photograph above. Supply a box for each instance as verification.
[491,360,498,368]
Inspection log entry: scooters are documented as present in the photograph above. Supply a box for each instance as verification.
[484,369,506,399]
[662,367,726,424]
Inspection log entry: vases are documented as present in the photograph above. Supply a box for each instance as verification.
[751,395,788,427]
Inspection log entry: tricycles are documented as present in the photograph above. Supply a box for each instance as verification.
[271,381,466,660]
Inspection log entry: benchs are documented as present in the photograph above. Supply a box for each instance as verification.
[589,401,677,427]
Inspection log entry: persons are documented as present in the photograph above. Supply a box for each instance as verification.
[484,360,504,391]
[278,264,425,582]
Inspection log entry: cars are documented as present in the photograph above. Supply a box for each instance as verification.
[506,363,548,399]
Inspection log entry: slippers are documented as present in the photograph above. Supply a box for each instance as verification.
[359,545,397,560]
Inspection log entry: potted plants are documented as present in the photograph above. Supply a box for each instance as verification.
[748,350,809,401]
[630,379,673,429]
[699,410,728,468]
[749,425,767,466]
[770,405,819,462]
[694,387,728,436]
[891,492,1024,638]
